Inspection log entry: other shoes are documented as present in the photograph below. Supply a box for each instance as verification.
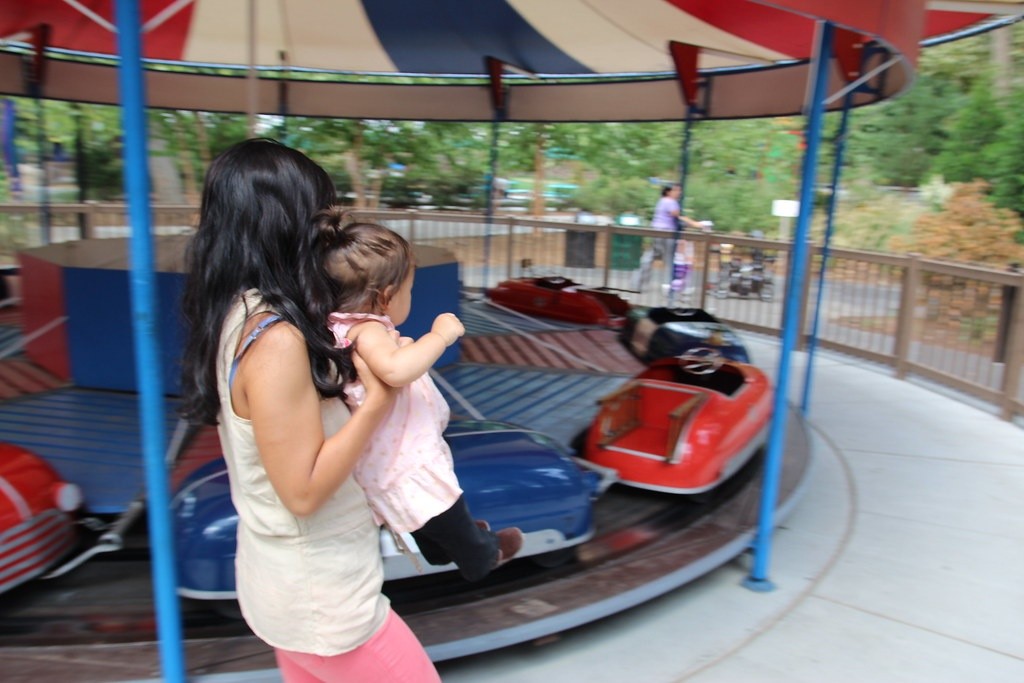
[474,520,523,570]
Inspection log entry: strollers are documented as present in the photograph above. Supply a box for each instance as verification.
[709,229,778,302]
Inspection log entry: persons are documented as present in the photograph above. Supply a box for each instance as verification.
[303,204,524,582]
[174,135,442,683]
[633,183,704,308]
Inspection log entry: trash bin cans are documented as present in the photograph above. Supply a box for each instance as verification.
[564,212,598,269]
[607,210,645,270]
